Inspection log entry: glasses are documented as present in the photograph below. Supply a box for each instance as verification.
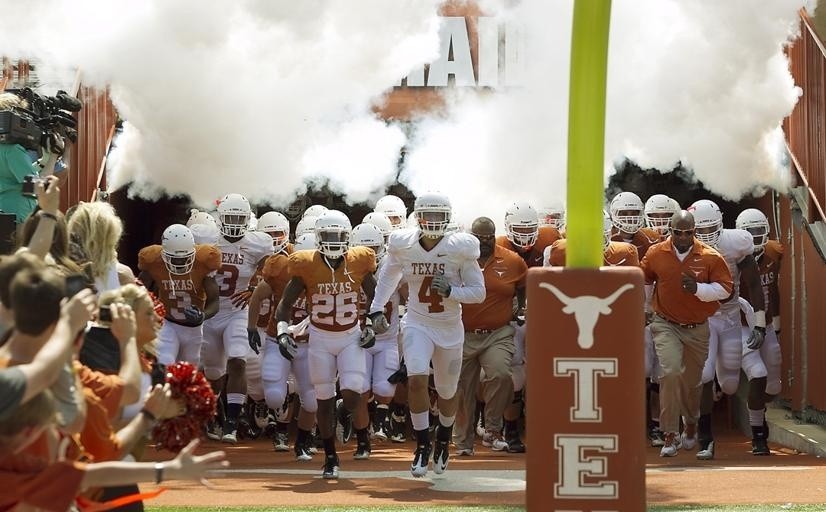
[471,232,495,240]
[672,228,695,236]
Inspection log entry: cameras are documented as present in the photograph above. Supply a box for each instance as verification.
[99,306,113,320]
[21,177,48,197]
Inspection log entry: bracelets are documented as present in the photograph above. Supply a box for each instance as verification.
[35,159,45,170]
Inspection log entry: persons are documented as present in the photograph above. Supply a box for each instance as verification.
[0,92,66,256]
[27,149,70,191]
[0,183,783,511]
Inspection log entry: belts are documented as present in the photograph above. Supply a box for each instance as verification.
[656,313,705,329]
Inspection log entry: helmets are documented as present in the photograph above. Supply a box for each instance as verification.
[505,197,565,248]
[602,191,771,252]
[160,194,451,276]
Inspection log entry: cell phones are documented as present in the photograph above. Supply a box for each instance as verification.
[65,276,84,297]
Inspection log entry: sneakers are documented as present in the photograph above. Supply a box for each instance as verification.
[650,420,714,459]
[750,426,770,455]
[713,378,721,402]
[455,411,525,457]
[411,419,449,477]
[203,381,292,450]
[323,451,338,480]
[296,421,323,460]
[337,399,406,458]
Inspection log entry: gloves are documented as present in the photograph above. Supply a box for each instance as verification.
[746,326,766,349]
[247,327,261,354]
[431,269,451,297]
[359,311,390,348]
[230,286,255,310]
[277,333,298,361]
[184,305,205,324]
